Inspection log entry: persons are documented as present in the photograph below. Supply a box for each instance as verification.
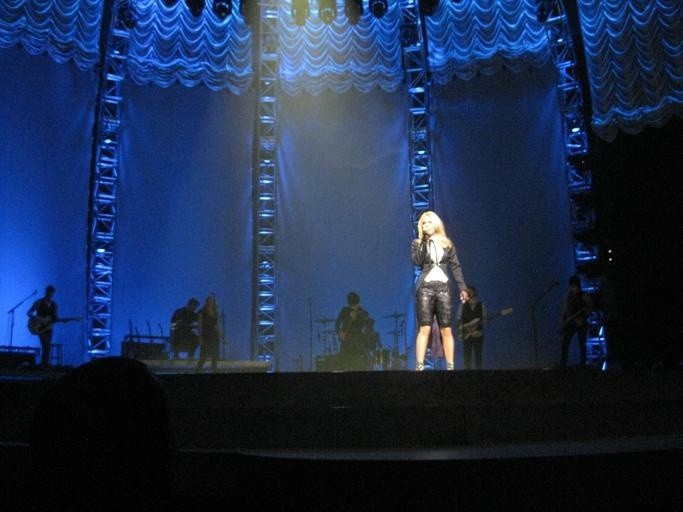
[409,210,471,372]
[25,284,58,367]
[169,296,200,361]
[455,284,489,370]
[332,292,369,373]
[361,318,383,359]
[194,295,227,372]
[555,274,593,369]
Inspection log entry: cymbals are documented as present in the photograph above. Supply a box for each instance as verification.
[323,330,337,334]
[383,314,405,318]
[315,319,334,323]
[387,330,400,333]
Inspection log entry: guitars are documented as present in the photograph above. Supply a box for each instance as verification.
[27,316,85,336]
[455,308,515,343]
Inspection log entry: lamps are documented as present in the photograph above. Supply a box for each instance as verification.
[369,0,387,19]
[316,0,338,26]
[343,0,363,24]
[212,0,231,21]
[288,0,310,25]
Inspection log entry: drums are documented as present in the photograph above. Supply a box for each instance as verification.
[324,352,345,371]
[374,347,406,366]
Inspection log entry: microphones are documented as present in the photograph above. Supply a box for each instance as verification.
[33,290,37,295]
[553,281,561,285]
[425,231,430,254]
[211,291,216,297]
[308,297,312,306]
